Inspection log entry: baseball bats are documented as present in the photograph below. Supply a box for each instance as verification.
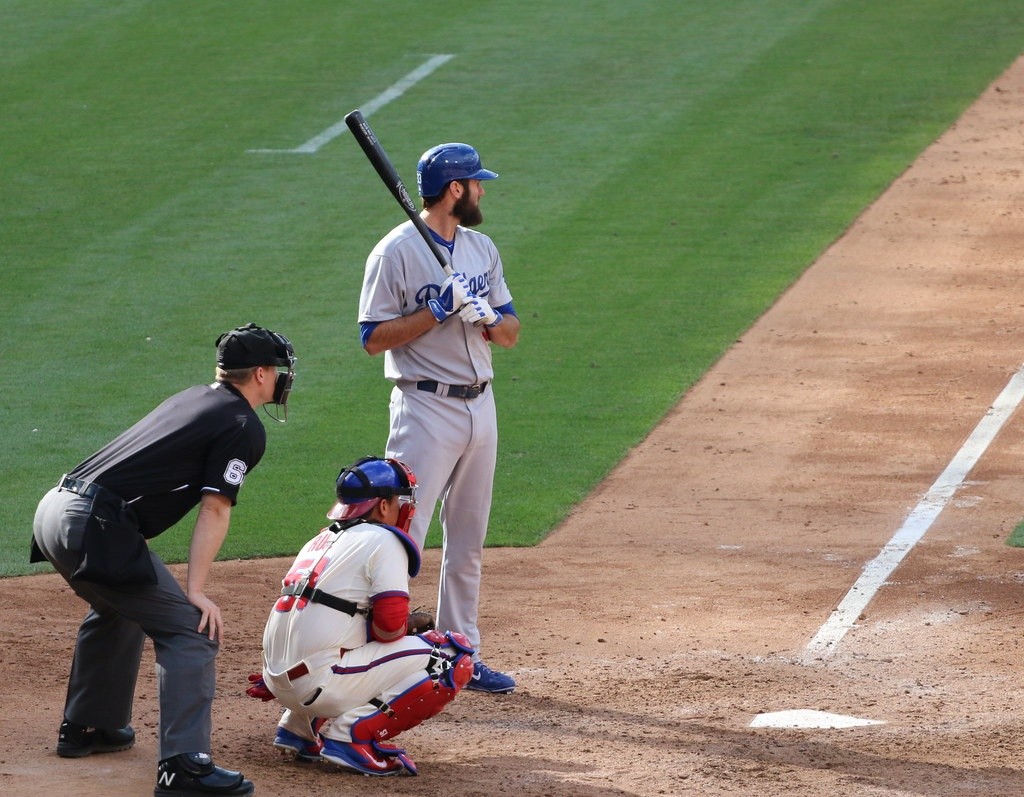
[341,108,489,330]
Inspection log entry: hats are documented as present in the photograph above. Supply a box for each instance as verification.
[216,324,293,370]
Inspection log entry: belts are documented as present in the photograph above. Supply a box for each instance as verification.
[56,478,98,496]
[416,378,486,400]
[287,647,345,680]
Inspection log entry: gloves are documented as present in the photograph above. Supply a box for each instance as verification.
[246,673,275,702]
[459,293,497,326]
[427,271,471,323]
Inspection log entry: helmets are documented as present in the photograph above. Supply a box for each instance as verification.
[326,457,412,523]
[416,143,499,197]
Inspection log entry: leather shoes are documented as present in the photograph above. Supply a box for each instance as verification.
[153,752,254,797]
[57,720,135,757]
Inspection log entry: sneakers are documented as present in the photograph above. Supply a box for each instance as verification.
[462,661,517,692]
[319,736,405,775]
[273,708,328,761]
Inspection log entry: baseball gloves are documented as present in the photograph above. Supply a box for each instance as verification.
[406,605,436,636]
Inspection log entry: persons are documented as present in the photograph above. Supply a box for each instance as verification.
[33,323,295,797]
[356,141,521,693]
[259,453,475,776]
